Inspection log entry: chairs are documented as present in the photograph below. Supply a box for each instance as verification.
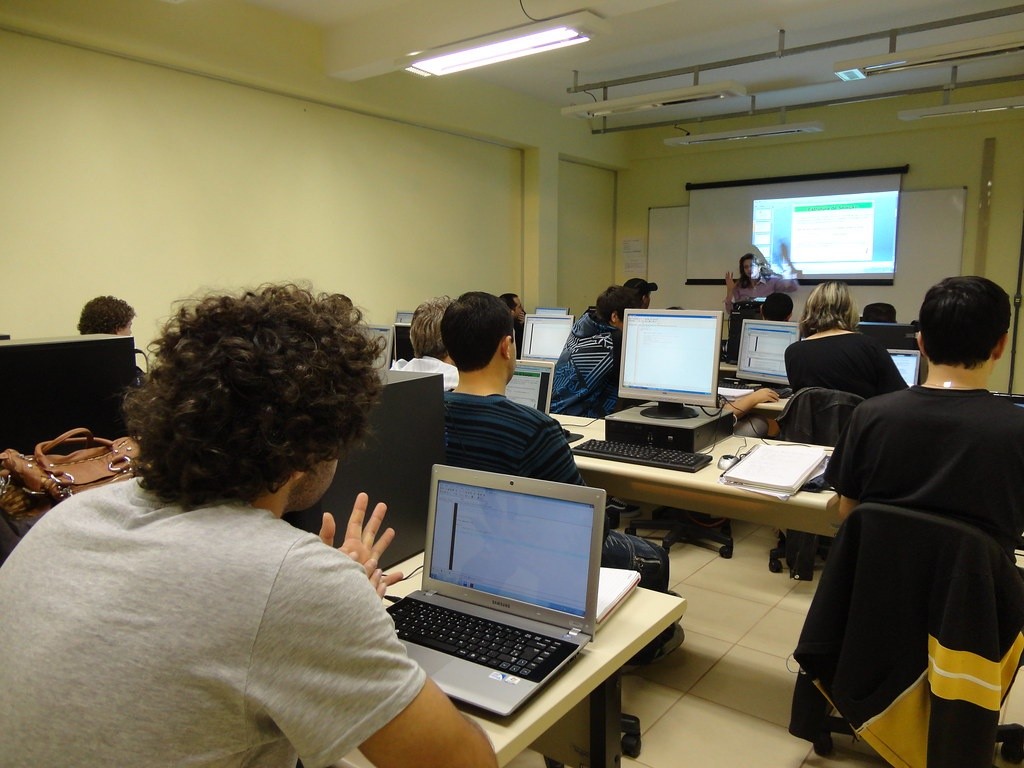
[812,503,1024,764]
[769,385,866,572]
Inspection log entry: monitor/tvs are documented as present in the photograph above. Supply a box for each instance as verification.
[619,308,724,419]
[854,321,921,389]
[736,319,805,398]
[363,307,574,415]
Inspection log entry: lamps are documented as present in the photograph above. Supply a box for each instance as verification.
[898,96,1024,121]
[561,81,746,118]
[393,11,612,77]
[833,30,1024,82]
[664,121,825,146]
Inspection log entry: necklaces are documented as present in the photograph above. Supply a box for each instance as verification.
[922,383,971,389]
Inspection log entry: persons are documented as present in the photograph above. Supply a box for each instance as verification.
[784,281,911,399]
[863,302,896,324]
[823,277,1024,667]
[323,293,354,320]
[499,293,526,359]
[76,296,146,389]
[549,285,641,418]
[623,278,658,309]
[0,277,499,768]
[722,241,799,320]
[390,294,459,392]
[441,291,686,674]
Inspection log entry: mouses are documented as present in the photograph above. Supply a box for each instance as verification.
[718,454,739,470]
[562,429,570,438]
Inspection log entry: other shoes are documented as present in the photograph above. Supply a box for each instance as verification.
[619,620,684,673]
[667,589,684,620]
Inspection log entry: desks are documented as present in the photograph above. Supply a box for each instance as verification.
[326,552,687,768]
[549,412,843,538]
[720,361,789,410]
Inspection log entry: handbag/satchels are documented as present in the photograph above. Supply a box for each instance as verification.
[0,427,151,560]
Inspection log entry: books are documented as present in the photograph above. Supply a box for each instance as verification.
[724,444,827,492]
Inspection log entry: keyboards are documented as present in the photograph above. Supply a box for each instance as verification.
[571,439,713,473]
[718,382,763,392]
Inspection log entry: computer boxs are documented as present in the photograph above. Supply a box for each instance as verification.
[727,301,764,364]
[604,402,734,453]
[0,333,139,459]
[280,368,445,572]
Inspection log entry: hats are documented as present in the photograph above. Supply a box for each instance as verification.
[623,278,659,297]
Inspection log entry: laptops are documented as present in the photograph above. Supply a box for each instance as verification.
[386,464,606,716]
